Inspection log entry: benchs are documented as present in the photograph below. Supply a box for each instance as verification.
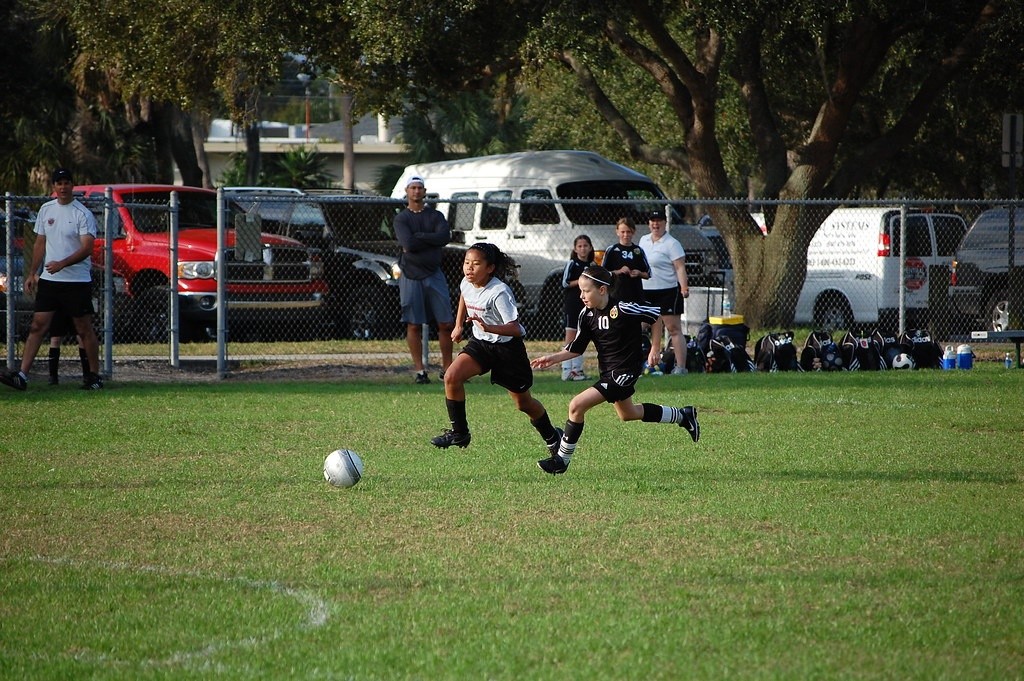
[972,330,1024,368]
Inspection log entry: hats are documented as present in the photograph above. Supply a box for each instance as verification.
[648,210,666,220]
[407,176,424,188]
[52,168,73,182]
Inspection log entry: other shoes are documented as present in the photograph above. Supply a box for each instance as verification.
[439,371,444,381]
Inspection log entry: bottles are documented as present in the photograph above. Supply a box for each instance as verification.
[723,295,731,317]
[391,261,401,280]
[1005,353,1011,368]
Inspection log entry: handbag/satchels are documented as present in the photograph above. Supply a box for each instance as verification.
[663,327,943,373]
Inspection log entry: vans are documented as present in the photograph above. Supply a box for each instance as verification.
[695,207,968,335]
[389,150,718,341]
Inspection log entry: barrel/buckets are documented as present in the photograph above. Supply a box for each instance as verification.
[956,344,976,368]
[943,345,955,369]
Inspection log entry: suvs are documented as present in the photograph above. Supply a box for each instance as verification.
[221,186,535,340]
[49,183,335,346]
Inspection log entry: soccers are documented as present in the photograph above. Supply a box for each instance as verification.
[892,353,916,369]
[324,449,363,488]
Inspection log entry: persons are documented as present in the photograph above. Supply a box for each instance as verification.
[561,207,690,382]
[530,266,701,476]
[0,168,103,391]
[394,176,470,386]
[431,242,564,460]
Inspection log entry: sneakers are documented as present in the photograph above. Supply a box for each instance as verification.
[672,366,688,375]
[79,377,102,390]
[679,405,700,442]
[547,426,564,456]
[415,371,430,383]
[1,373,27,391]
[561,370,592,381]
[537,454,571,475]
[430,430,471,449]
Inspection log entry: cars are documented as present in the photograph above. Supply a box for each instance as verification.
[0,209,135,343]
[945,206,1024,343]
